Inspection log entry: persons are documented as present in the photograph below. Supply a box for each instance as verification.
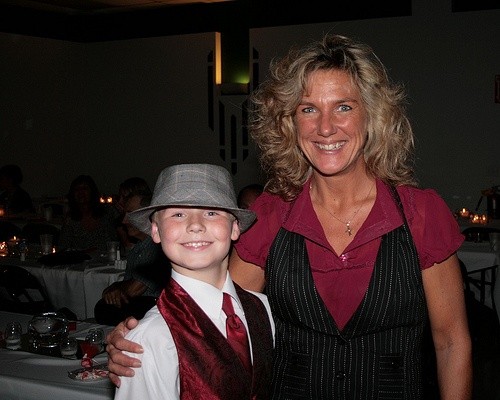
[114,164,278,400]
[0,163,272,325]
[106,36,473,400]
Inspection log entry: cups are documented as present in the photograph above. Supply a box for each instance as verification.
[40,234,53,254]
[489,233,499,253]
[59,337,78,356]
[107,241,118,262]
[4,322,22,345]
[88,329,104,344]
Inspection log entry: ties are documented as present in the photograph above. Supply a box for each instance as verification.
[222,293,253,390]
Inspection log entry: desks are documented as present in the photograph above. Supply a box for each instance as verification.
[8,249,130,320]
[0,312,123,399]
[457,211,500,314]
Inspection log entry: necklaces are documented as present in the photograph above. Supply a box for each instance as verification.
[309,181,378,237]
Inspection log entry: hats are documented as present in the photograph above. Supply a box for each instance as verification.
[126,164,258,233]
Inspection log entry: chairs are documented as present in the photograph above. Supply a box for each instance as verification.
[0,203,157,327]
[422,264,500,399]
